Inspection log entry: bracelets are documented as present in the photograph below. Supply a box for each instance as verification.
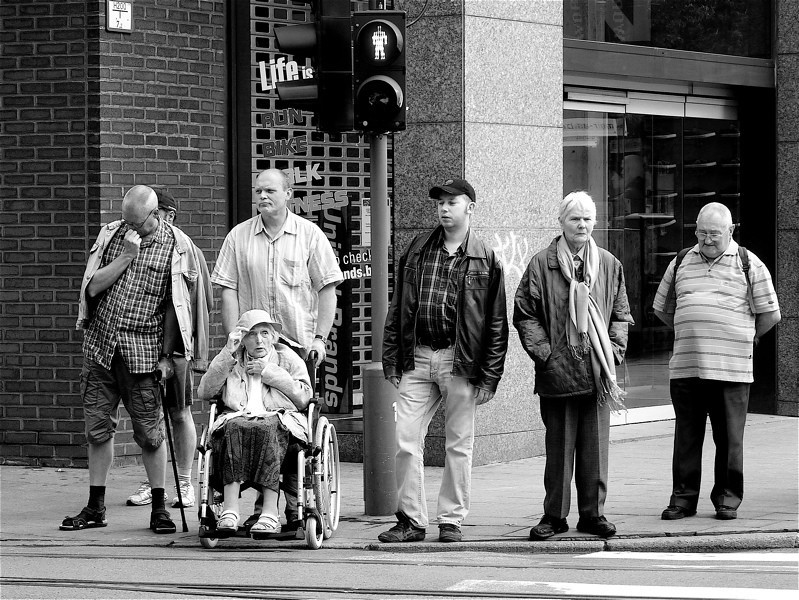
[161,354,173,359]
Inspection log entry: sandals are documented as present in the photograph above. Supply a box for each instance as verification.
[217,510,241,533]
[150,509,176,534]
[58,506,108,530]
[250,514,282,533]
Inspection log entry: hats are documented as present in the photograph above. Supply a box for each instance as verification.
[154,189,176,212]
[429,179,476,203]
[234,309,282,343]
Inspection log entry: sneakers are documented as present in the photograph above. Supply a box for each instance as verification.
[577,515,617,537]
[661,504,696,518]
[529,514,569,539]
[714,505,738,519]
[438,523,462,542]
[172,480,195,508]
[378,510,426,542]
[126,479,168,506]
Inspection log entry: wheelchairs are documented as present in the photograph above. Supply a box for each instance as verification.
[197,350,341,550]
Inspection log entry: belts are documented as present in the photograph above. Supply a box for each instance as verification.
[417,337,455,351]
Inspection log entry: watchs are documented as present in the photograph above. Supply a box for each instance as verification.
[314,334,327,344]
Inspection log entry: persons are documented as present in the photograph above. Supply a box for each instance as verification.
[197,309,313,534]
[377,178,509,544]
[125,184,197,508]
[652,203,782,521]
[58,183,214,536]
[210,168,344,532]
[512,192,636,540]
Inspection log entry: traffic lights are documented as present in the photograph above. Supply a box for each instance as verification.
[353,9,406,131]
[274,0,354,133]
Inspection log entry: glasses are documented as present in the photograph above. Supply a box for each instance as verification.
[695,227,729,241]
[120,209,154,231]
